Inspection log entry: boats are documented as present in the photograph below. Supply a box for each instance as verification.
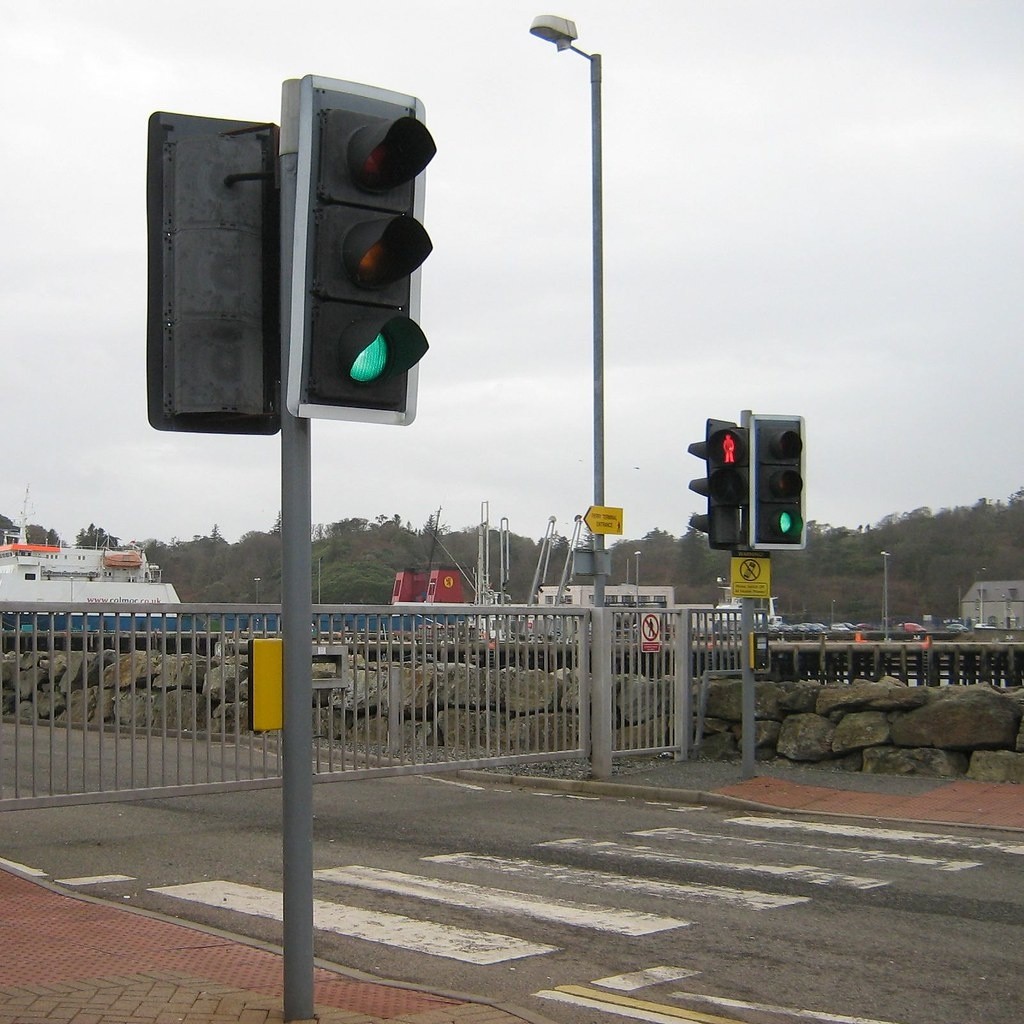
[0,481,185,634]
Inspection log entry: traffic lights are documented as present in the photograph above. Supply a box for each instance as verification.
[749,414,807,550]
[688,419,750,550]
[285,75,438,426]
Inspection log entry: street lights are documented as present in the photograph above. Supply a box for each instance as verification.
[529,15,607,607]
[880,550,891,641]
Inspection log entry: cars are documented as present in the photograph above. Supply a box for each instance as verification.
[831,623,850,631]
[844,623,860,631]
[946,623,968,632]
[856,624,871,630]
[767,622,823,633]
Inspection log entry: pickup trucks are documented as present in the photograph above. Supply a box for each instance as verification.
[900,623,926,633]
